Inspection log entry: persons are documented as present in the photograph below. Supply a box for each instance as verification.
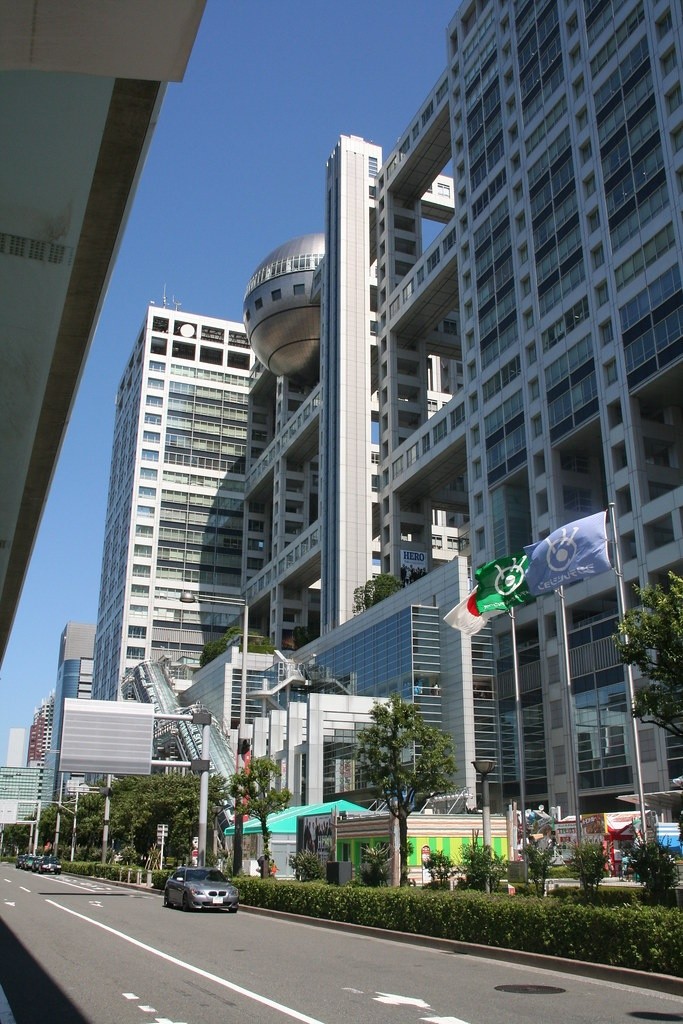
[621,851,634,882]
[304,817,332,853]
[271,859,280,879]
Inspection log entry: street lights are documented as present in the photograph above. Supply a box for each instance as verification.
[471,760,496,895]
[180,592,250,874]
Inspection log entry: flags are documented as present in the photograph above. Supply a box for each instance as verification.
[474,550,538,614]
[443,584,507,636]
[522,510,612,597]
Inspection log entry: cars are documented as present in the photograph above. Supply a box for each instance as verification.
[16,855,61,875]
[163,868,239,913]
